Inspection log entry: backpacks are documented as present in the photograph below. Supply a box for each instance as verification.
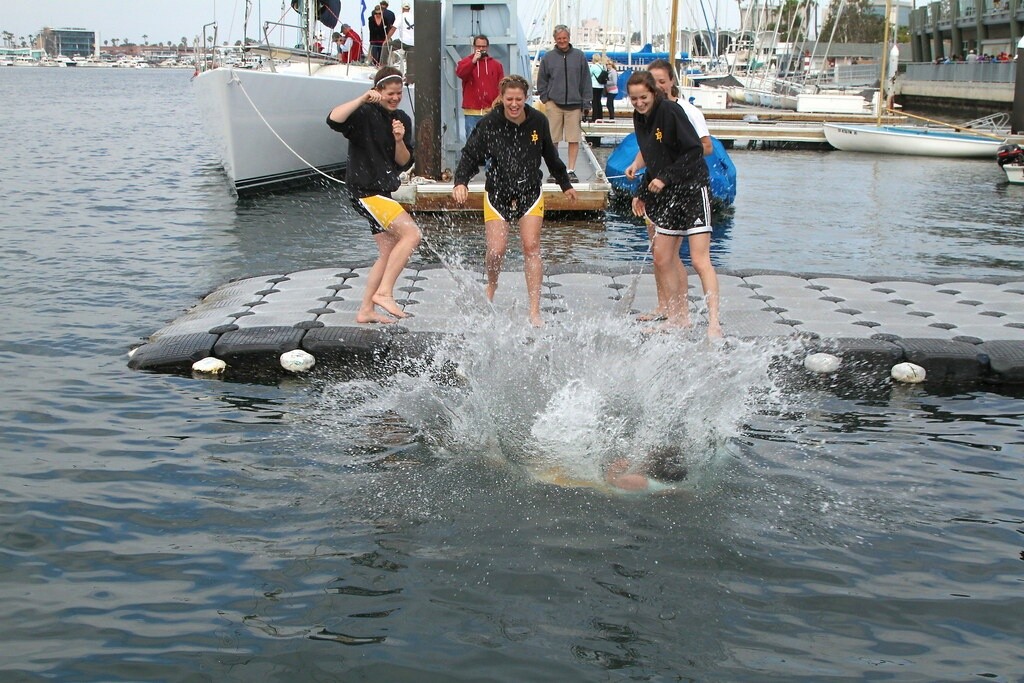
[593,64,608,84]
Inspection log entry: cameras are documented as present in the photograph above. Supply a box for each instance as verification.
[479,50,487,57]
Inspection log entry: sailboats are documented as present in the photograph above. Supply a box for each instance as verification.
[37,32,73,68]
[518,0,728,88]
[684,0,866,113]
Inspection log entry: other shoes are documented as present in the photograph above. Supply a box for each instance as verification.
[604,119,615,123]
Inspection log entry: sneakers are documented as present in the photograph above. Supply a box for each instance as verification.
[567,172,579,183]
[547,173,557,183]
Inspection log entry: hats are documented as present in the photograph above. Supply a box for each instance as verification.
[332,32,341,42]
[340,24,348,33]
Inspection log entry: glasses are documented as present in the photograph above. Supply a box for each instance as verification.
[375,13,381,15]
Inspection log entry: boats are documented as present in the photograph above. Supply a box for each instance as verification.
[0,53,36,67]
[73,54,149,68]
[191,0,416,198]
[996,144,1024,184]
[822,122,1024,158]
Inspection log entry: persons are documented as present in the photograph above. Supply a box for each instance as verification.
[604,60,618,124]
[627,72,723,340]
[330,24,361,63]
[366,0,395,66]
[626,59,713,329]
[452,74,577,329]
[379,2,415,84]
[934,49,1018,83]
[607,448,689,491]
[455,34,504,183]
[589,53,605,123]
[325,66,423,324]
[536,25,592,183]
